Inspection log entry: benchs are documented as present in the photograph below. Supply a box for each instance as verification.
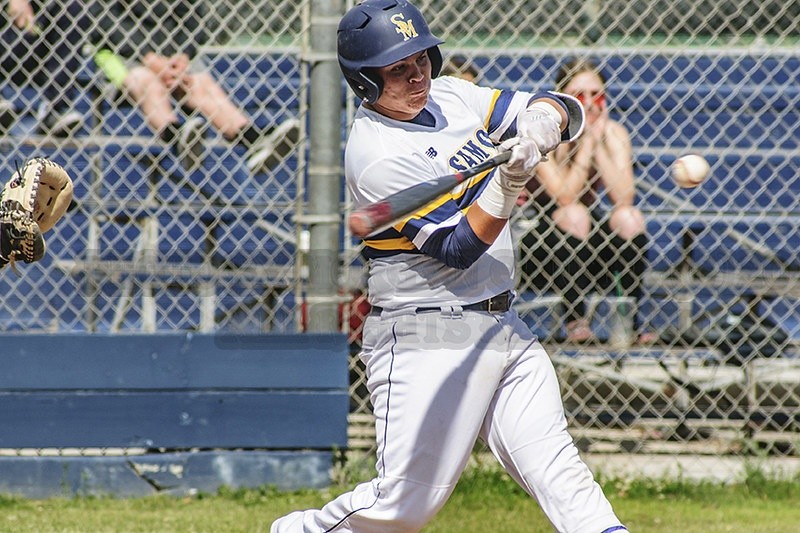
[0,52,800,351]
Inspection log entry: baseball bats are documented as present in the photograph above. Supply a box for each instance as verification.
[346,150,510,238]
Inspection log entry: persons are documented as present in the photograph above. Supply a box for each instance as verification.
[440,53,480,85]
[521,55,660,350]
[92,1,300,171]
[1,1,84,139]
[270,0,632,532]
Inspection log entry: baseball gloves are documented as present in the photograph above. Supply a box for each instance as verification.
[0,158,73,270]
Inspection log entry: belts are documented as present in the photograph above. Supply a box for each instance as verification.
[372,291,512,315]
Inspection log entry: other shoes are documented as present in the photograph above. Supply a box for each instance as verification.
[175,117,206,173]
[248,125,300,176]
[568,319,595,342]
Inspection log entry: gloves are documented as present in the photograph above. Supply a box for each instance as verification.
[515,103,561,156]
[497,136,542,193]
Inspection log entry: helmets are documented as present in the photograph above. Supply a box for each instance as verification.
[336,1,443,104]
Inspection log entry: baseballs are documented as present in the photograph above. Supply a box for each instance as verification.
[672,153,710,187]
